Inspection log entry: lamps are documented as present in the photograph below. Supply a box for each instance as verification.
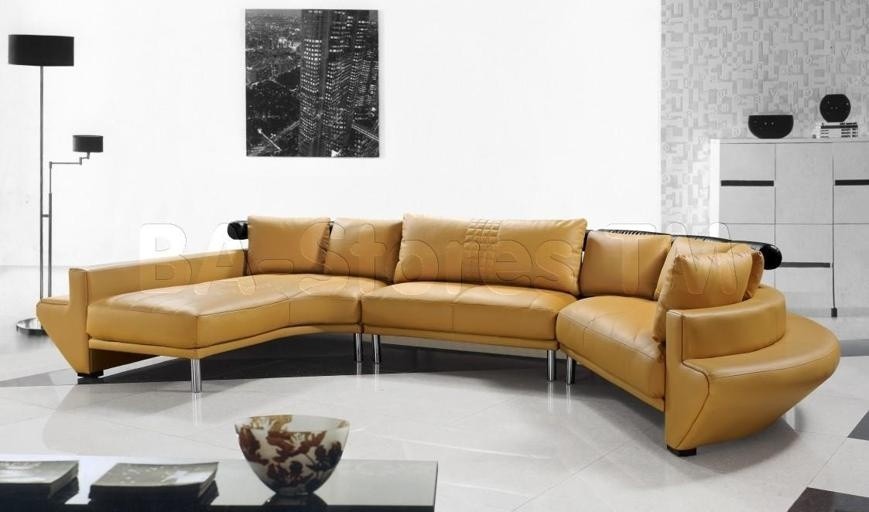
[6,34,104,336]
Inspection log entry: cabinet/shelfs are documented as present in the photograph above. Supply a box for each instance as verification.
[706,134,869,342]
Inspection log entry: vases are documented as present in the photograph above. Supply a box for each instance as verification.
[820,93,851,122]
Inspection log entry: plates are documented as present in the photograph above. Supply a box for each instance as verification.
[0,460,220,501]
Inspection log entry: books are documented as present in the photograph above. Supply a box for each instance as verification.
[88,461,221,504]
[0,460,80,502]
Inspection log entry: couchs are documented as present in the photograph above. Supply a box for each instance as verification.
[34,220,841,459]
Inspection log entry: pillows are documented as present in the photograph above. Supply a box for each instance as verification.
[245,214,589,298]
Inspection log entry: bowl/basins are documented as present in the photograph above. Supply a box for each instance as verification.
[235,414,351,497]
[747,114,794,138]
